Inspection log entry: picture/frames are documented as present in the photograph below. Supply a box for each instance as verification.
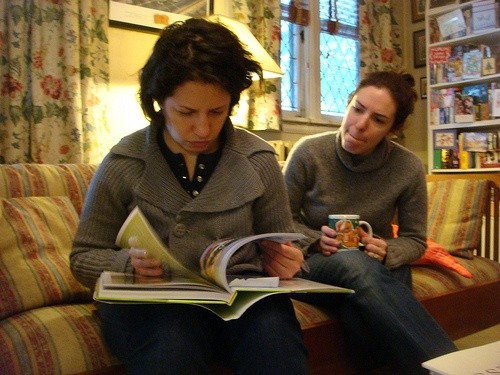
[413,29,426,68]
[411,0,424,23]
[420,77,427,99]
[481,56,496,76]
[434,130,456,149]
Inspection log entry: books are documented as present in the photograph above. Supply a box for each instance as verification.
[93,205,356,321]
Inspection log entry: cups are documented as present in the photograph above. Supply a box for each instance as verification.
[329,215,373,252]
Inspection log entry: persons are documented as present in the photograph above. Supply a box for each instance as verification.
[69,19,311,374]
[281,72,458,375]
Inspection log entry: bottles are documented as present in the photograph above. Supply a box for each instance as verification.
[451,153,459,168]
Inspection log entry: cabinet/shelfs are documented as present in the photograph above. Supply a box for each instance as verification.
[424,0,500,174]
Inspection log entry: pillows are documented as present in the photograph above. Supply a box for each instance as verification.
[0,194,95,319]
[390,224,472,279]
[425,179,490,258]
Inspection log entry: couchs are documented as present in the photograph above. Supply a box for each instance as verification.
[0,163,500,375]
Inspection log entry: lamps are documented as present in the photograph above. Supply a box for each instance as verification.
[207,16,283,82]
